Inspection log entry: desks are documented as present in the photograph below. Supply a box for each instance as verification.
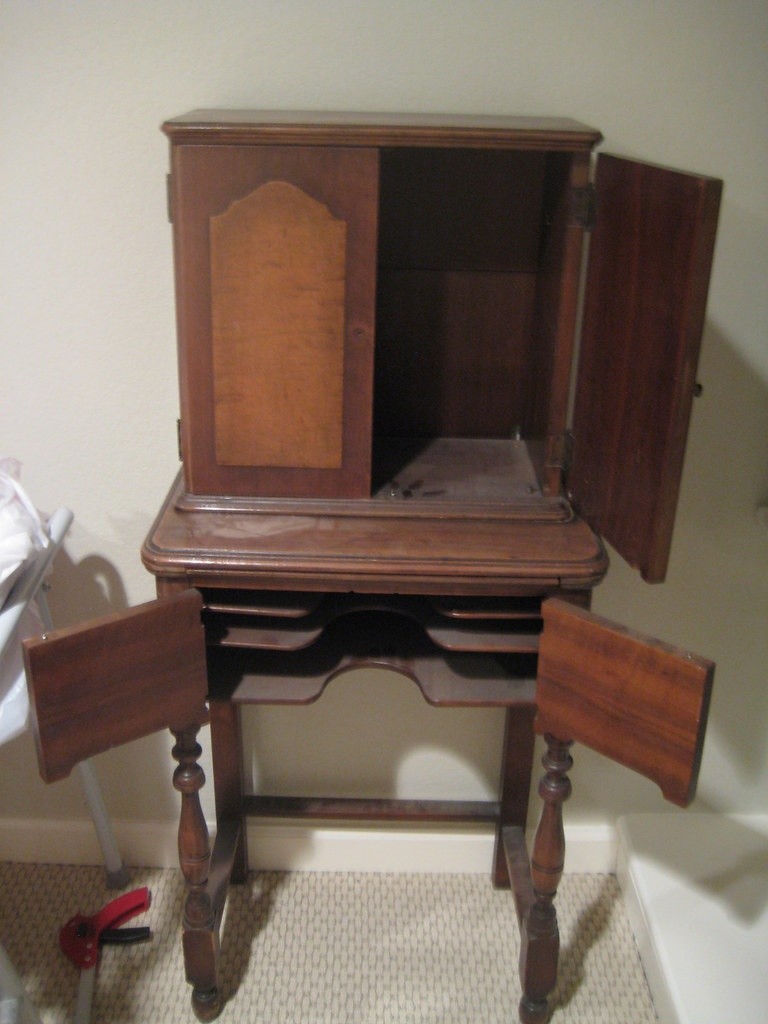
[21,473,723,1024]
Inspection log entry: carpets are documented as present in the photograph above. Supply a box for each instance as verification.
[0,858,660,1024]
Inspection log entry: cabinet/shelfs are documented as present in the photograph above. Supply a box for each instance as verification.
[159,106,727,587]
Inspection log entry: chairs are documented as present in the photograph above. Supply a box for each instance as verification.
[0,471,134,889]
